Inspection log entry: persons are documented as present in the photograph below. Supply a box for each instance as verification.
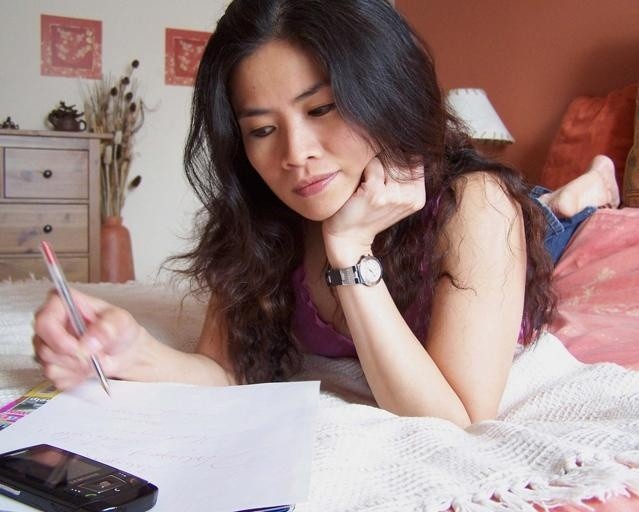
[30,2,622,433]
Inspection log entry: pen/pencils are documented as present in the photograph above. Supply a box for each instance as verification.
[233,505,294,512]
[41,242,114,399]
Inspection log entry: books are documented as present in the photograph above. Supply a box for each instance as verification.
[0,377,321,512]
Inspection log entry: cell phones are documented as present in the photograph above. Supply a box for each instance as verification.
[0,444,159,512]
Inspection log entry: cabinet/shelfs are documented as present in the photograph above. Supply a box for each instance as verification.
[1,126,119,284]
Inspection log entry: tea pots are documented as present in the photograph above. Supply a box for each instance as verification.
[48,109,88,131]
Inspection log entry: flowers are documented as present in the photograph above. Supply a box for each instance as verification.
[86,58,154,217]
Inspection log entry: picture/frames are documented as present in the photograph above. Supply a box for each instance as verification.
[163,27,214,88]
[39,13,104,81]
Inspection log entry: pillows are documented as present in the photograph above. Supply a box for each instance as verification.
[541,80,638,207]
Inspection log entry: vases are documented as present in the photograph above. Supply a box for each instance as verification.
[100,216,138,284]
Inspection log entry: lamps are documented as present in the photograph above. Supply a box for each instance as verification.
[444,87,518,153]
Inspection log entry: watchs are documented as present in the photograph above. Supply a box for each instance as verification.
[323,252,386,289]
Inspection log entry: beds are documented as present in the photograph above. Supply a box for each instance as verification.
[1,195,639,511]
